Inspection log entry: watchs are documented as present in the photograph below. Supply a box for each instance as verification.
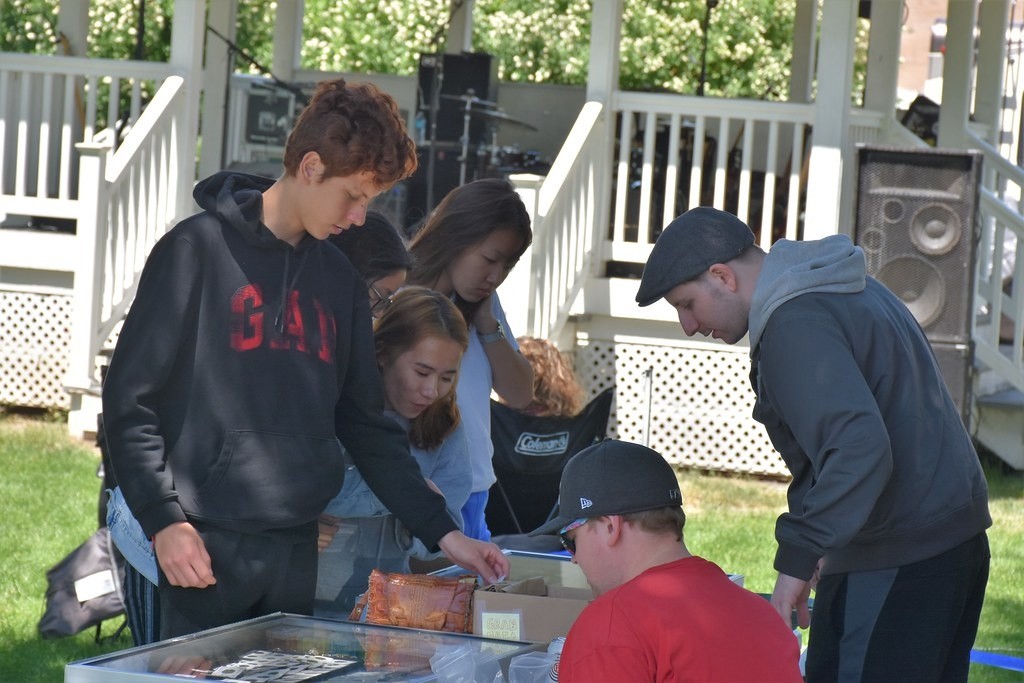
[475,320,505,344]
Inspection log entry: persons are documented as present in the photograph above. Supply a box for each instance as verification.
[526,438,804,683]
[106,211,411,645]
[101,80,511,641]
[498,335,579,419]
[635,207,993,683]
[395,177,535,542]
[312,287,473,611]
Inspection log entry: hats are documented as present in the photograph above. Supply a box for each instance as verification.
[635,205,756,307]
[525,437,686,538]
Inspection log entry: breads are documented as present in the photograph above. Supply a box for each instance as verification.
[367,568,475,635]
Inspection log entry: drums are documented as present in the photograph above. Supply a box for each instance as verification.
[401,138,554,227]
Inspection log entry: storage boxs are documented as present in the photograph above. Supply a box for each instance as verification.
[472,578,596,644]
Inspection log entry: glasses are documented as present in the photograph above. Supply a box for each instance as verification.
[369,285,391,320]
[556,518,592,556]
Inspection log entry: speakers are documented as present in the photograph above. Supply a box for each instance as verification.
[850,143,983,436]
[406,51,499,240]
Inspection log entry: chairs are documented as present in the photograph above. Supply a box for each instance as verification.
[486,385,616,539]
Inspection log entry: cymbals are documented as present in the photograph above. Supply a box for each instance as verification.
[444,86,502,112]
[447,104,539,132]
